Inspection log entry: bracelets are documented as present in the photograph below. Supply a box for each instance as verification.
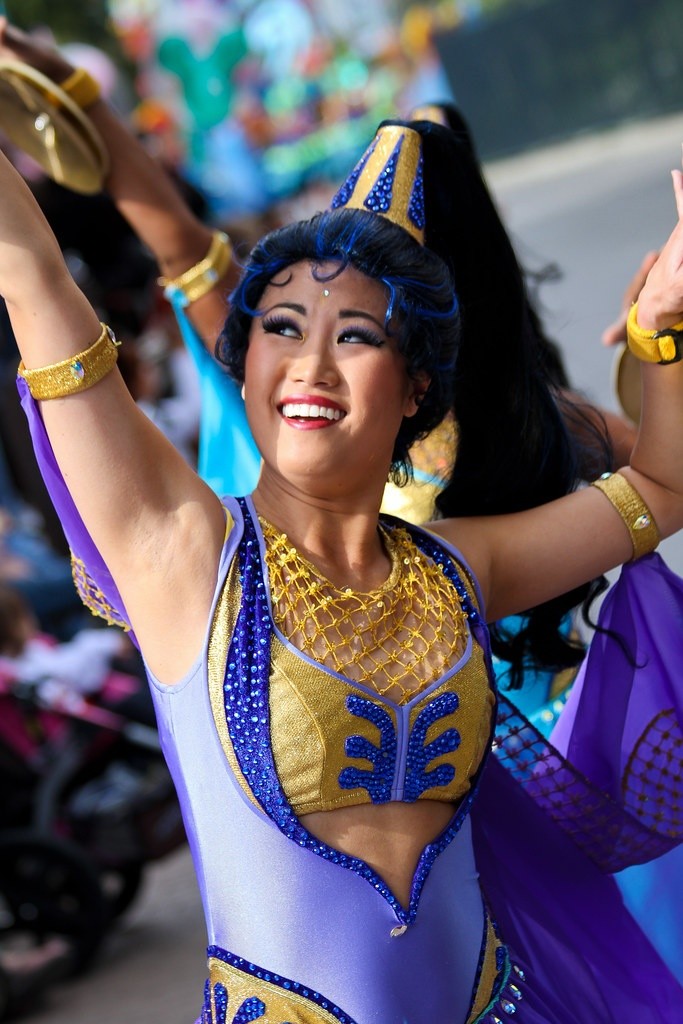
[626,303,682,363]
[60,69,105,113]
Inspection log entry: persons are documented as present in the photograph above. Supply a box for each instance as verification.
[0,18,661,740]
[1,120,683,1024]
